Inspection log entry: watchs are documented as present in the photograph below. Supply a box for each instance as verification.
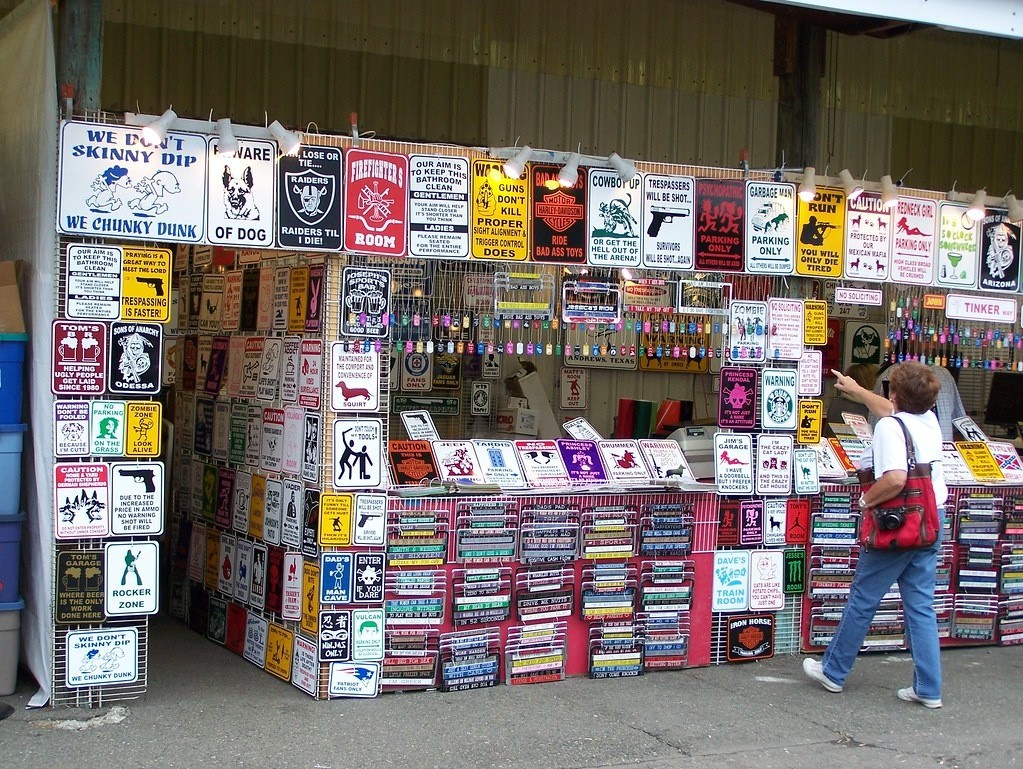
[859,497,866,508]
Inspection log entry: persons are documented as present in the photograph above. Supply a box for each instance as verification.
[803,360,948,708]
[824,364,876,438]
[869,322,966,441]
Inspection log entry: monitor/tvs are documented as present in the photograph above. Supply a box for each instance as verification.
[984,371,1023,439]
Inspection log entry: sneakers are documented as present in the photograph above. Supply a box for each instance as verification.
[803,657,842,692]
[897,686,942,708]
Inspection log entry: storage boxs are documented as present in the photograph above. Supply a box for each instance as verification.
[0,333,31,696]
[0,258,29,334]
[495,356,562,441]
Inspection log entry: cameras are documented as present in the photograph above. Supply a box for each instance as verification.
[876,507,907,531]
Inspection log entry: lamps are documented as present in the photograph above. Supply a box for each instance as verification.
[558,152,583,187]
[880,175,900,208]
[839,168,864,200]
[797,167,816,202]
[1003,194,1023,222]
[607,152,638,182]
[216,118,239,154]
[503,145,533,179]
[269,120,301,156]
[141,109,177,144]
[967,190,987,221]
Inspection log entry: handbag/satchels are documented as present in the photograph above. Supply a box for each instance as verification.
[857,415,939,551]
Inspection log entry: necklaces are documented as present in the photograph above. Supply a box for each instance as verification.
[344,257,784,359]
[884,284,1023,371]
